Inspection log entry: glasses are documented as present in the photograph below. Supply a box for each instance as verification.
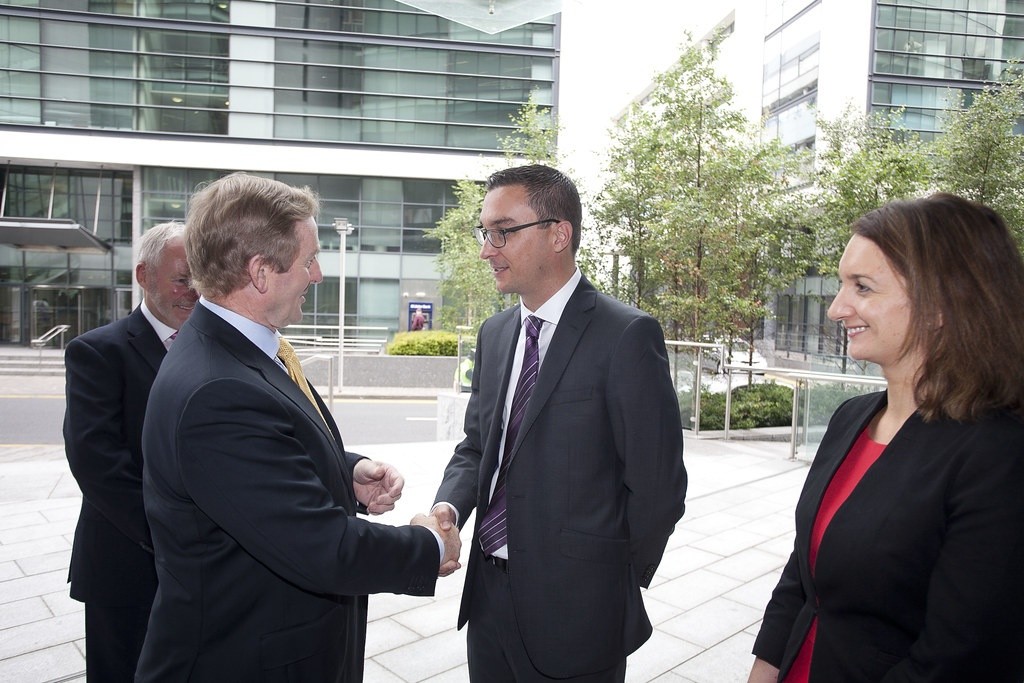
[475,219,560,248]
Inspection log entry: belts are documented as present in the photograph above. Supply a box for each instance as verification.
[488,556,510,575]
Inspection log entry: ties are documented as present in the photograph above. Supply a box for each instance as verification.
[279,336,329,434]
[475,315,546,559]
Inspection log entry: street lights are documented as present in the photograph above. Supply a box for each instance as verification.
[331,217,356,393]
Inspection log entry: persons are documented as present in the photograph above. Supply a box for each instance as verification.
[63,220,201,683]
[746,193,1024,683]
[411,308,425,331]
[428,164,688,683]
[131,172,463,683]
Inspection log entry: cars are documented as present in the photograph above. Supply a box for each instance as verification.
[700,334,768,375]
